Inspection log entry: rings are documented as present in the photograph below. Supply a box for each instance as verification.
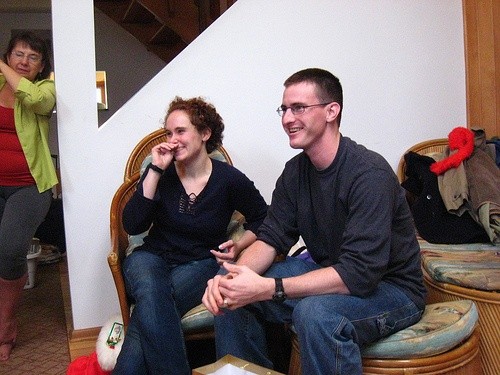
[224,297,228,307]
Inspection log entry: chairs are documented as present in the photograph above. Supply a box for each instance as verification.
[107,128,246,340]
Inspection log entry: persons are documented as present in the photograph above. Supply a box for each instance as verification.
[202,68,428,375]
[111,96,269,375]
[0,32,58,360]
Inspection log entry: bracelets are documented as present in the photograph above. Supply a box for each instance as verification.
[147,163,164,174]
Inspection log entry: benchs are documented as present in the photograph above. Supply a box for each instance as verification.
[397,138,500,375]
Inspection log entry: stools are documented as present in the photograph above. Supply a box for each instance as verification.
[288,300,480,375]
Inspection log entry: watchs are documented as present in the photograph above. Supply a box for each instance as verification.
[271,278,287,305]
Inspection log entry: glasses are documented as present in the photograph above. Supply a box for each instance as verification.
[12,50,44,66]
[276,102,330,117]
